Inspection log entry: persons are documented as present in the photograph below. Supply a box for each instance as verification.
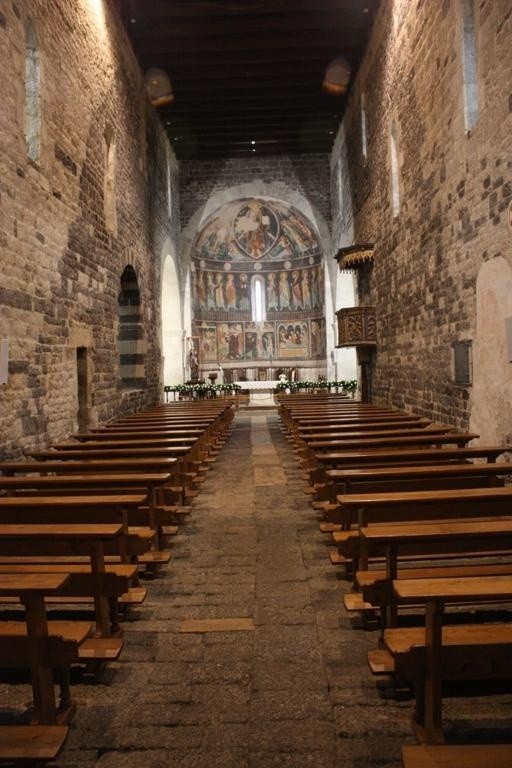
[196,268,319,312]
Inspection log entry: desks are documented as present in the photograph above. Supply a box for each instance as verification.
[304,480,511,564]
[344,518,511,740]
[3,399,233,488]
[277,389,510,479]
[1,490,200,577]
[3,564,147,719]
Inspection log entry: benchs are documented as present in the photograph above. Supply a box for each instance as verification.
[0,724,71,765]
[399,741,510,768]
